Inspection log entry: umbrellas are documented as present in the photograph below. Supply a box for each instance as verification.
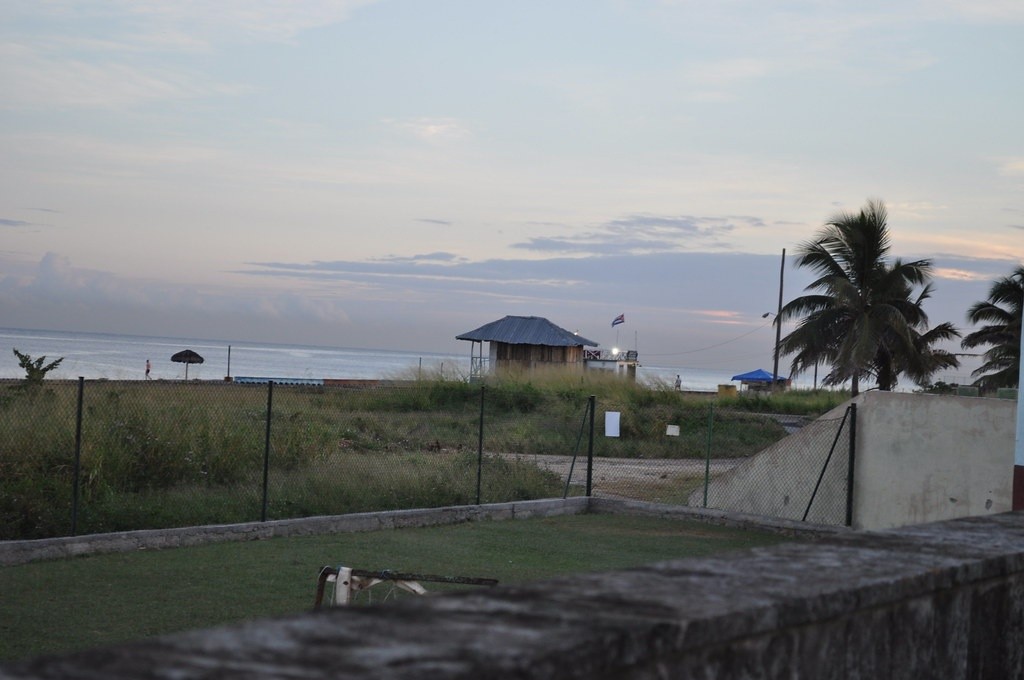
[171,349,204,381]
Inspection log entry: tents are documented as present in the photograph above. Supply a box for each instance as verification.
[731,368,789,393]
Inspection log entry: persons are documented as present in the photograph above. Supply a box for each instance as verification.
[675,374,681,391]
[145,359,151,379]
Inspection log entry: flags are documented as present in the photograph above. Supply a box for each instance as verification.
[613,313,625,326]
